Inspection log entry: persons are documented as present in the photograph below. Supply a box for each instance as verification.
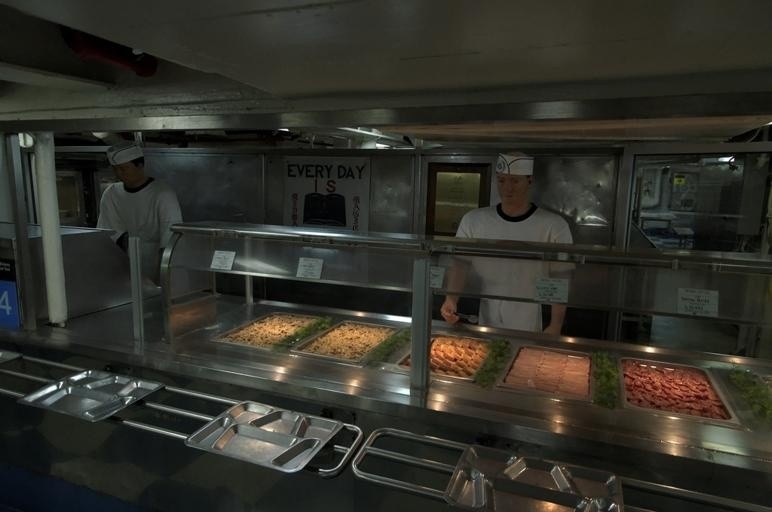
[441,151,574,335]
[96,142,183,286]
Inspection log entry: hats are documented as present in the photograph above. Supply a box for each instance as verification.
[107,143,144,166]
[495,151,535,176]
